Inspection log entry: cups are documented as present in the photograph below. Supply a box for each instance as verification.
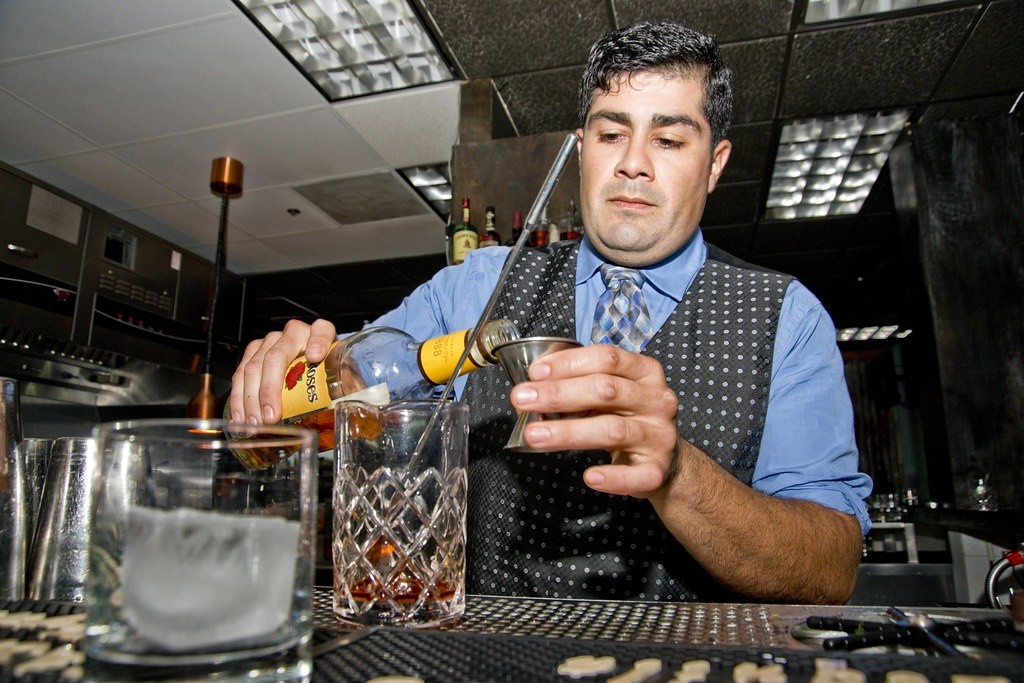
[24,437,101,607]
[331,396,469,626]
[94,430,154,527]
[76,415,316,683]
[0,377,33,608]
[490,336,586,452]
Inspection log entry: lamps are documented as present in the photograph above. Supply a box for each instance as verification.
[186,156,244,434]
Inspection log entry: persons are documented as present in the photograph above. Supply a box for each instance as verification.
[231,18,872,606]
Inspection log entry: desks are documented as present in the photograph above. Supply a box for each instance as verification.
[305,585,1024,682]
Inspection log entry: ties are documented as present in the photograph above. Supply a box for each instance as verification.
[590,262,655,355]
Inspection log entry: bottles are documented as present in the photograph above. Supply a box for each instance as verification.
[478,205,501,249]
[452,197,480,267]
[221,320,522,472]
[505,209,532,248]
[559,195,585,241]
